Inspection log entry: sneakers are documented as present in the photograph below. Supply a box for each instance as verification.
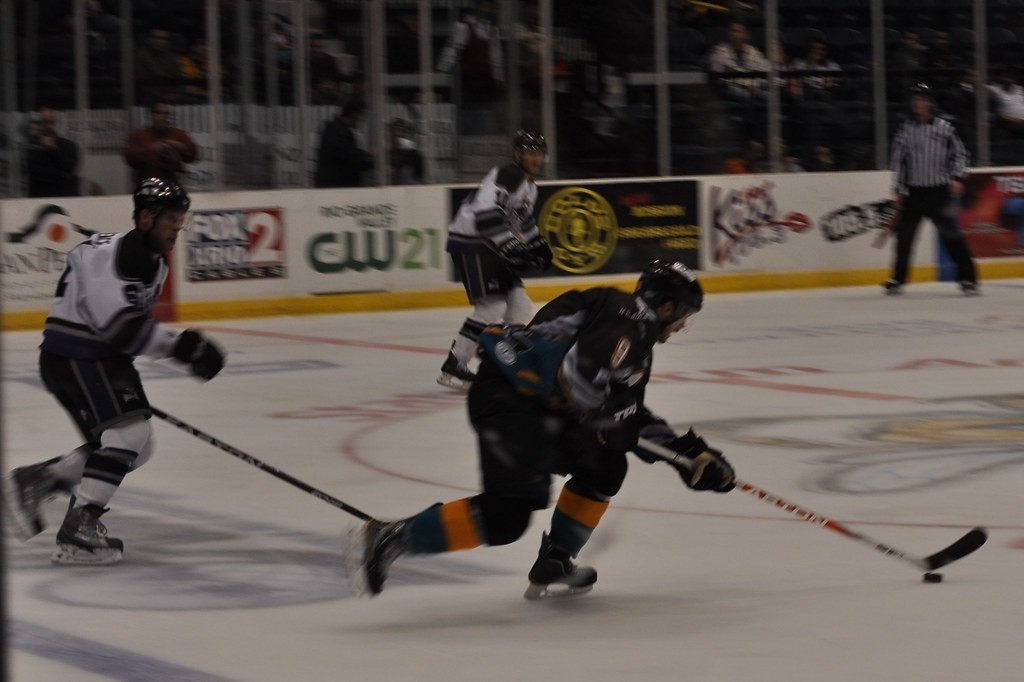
[880,276,899,295]
[51,493,124,564]
[525,529,597,601]
[961,278,978,297]
[0,456,65,542]
[436,340,478,390]
[348,502,444,601]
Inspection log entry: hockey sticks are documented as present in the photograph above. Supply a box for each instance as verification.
[637,435,987,571]
[139,404,444,526]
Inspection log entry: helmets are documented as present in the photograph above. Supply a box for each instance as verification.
[513,127,547,153]
[908,79,941,115]
[135,166,190,214]
[642,249,703,313]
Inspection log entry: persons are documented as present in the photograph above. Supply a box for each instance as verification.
[881,82,979,296]
[669,0,860,172]
[342,258,738,603]
[889,29,1024,140]
[713,177,784,263]
[0,174,225,564]
[120,101,202,194]
[437,128,554,389]
[437,6,624,138]
[318,96,426,189]
[10,0,365,104]
[18,107,82,197]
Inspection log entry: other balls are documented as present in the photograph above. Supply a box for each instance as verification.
[923,573,943,584]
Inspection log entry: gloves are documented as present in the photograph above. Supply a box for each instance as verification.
[596,379,640,453]
[174,332,223,381]
[667,427,737,493]
[498,237,530,270]
[527,233,553,272]
